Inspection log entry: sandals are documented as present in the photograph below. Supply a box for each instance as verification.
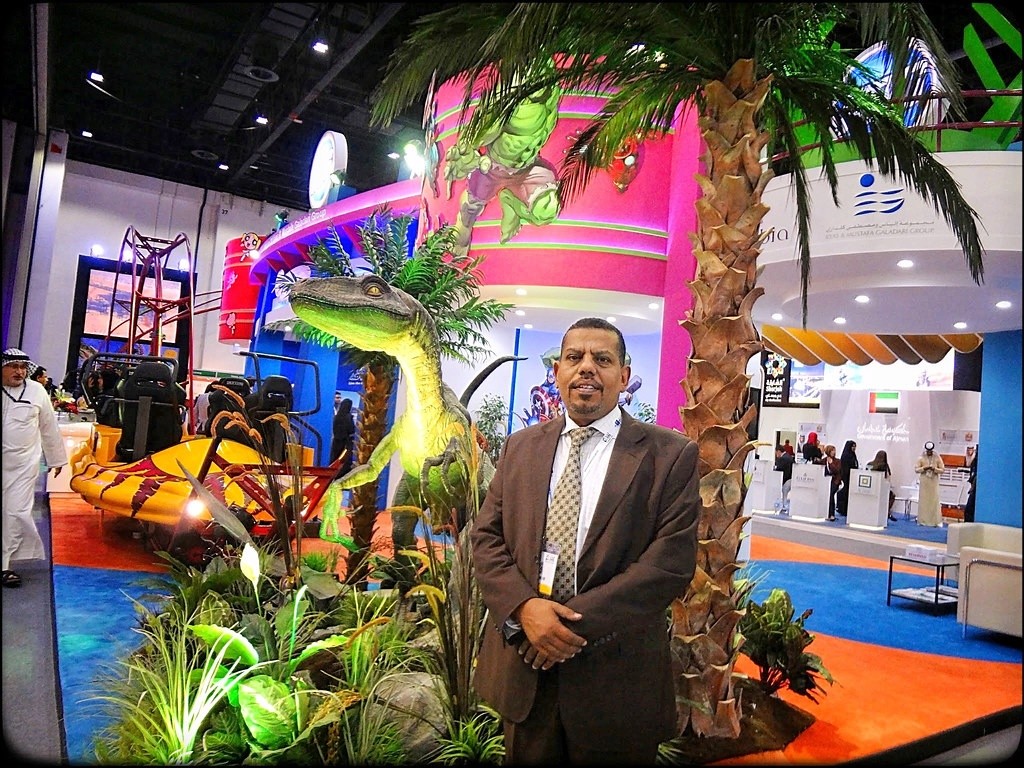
[2,570,21,588]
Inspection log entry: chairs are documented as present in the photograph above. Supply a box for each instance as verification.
[940,482,966,522]
[93,362,294,462]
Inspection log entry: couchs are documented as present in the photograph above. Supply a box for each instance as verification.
[946,522,1023,640]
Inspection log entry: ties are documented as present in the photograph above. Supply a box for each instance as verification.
[542,427,598,605]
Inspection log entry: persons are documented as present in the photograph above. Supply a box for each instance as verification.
[836,441,858,517]
[963,444,979,522]
[30,366,122,428]
[0,347,69,587]
[469,317,704,768]
[914,441,945,528]
[865,450,898,522]
[187,380,220,435]
[803,432,842,522]
[328,392,359,481]
[775,439,795,502]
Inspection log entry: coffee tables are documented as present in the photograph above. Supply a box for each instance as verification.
[886,554,961,617]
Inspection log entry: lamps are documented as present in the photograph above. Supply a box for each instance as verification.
[274,207,291,221]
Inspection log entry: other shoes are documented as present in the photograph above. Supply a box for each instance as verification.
[889,514,897,521]
[829,516,835,521]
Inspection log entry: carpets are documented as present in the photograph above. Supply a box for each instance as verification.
[774,510,948,543]
[734,559,1023,665]
